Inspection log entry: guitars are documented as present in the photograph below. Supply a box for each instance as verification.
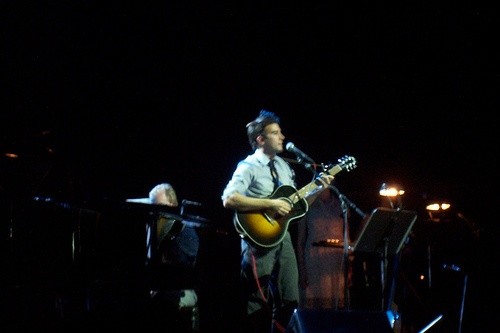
[234,155,357,250]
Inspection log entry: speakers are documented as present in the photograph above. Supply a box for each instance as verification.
[286,308,395,333]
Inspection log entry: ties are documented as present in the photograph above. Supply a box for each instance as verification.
[268,160,279,195]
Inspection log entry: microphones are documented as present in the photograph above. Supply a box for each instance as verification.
[286,142,315,163]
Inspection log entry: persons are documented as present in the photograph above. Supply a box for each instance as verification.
[127,181,198,333]
[221,115,334,332]
[298,188,348,331]
